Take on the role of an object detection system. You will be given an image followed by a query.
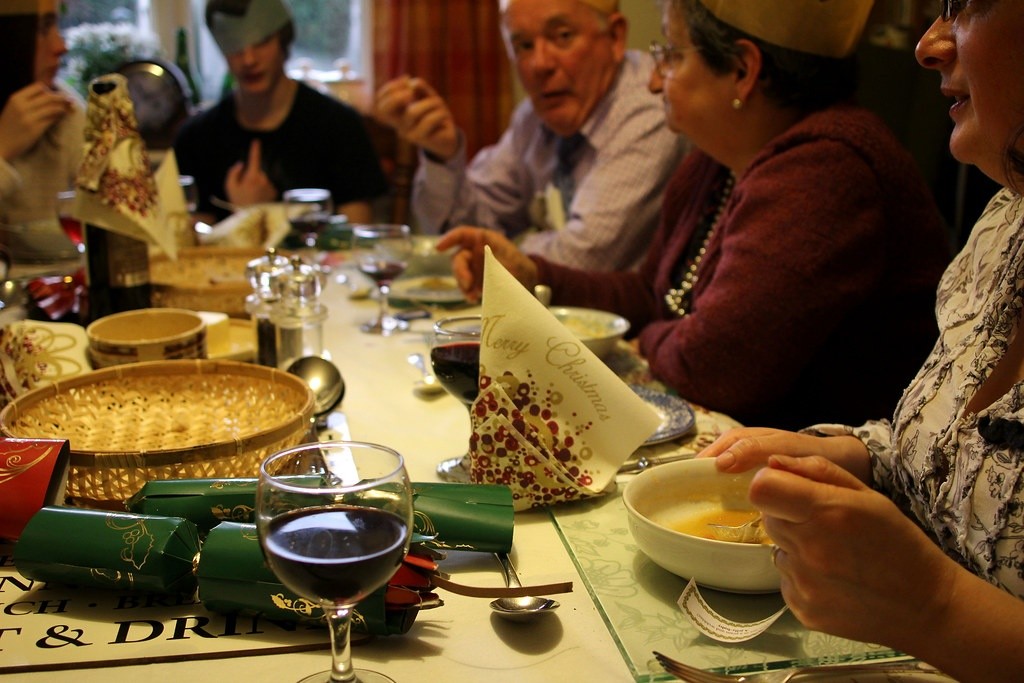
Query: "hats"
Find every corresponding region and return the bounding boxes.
[702,1,877,59]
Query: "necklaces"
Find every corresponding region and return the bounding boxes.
[664,174,740,319]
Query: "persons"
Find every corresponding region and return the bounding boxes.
[377,0,700,274]
[0,0,89,264]
[435,0,954,434]
[693,0,1024,683]
[171,0,388,232]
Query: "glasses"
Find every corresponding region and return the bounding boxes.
[941,0,968,22]
[649,39,742,78]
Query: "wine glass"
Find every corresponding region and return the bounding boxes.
[431,316,482,482]
[351,224,411,333]
[284,190,330,271]
[255,442,414,683]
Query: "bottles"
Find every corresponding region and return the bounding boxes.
[85,79,154,318]
[244,250,328,430]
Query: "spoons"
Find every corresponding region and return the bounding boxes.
[707,517,769,544]
[286,356,345,414]
[490,552,560,623]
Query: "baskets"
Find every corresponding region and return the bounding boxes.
[148,252,304,316]
[1,358,316,512]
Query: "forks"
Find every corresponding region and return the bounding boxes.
[651,650,940,683]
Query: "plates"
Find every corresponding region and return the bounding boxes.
[630,386,694,447]
[371,275,466,305]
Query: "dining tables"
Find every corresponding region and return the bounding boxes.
[0,236,956,683]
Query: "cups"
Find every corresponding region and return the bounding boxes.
[180,174,198,215]
[54,192,84,251]
[86,307,207,370]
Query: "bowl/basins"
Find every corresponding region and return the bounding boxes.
[545,306,631,361]
[622,459,783,595]
[377,236,459,274]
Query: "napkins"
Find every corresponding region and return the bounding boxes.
[472,244,664,513]
[0,321,100,405]
[74,75,175,260]
